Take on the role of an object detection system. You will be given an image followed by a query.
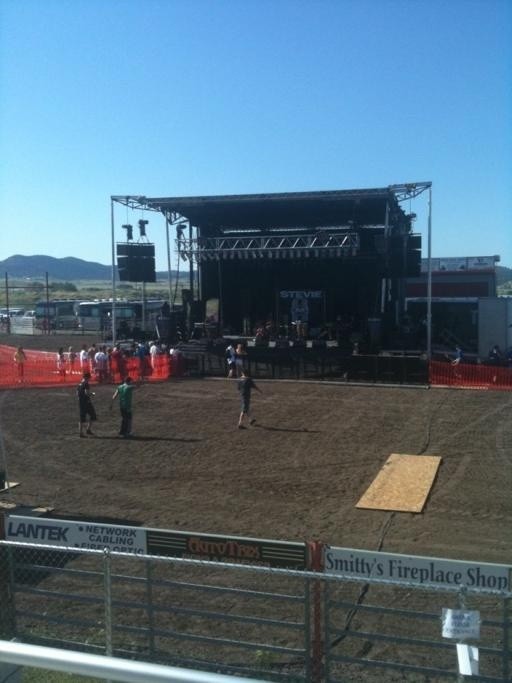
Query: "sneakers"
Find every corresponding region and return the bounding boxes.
[239,425,247,429]
[250,419,256,425]
[80,432,87,438]
[86,430,94,434]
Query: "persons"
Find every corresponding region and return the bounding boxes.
[110,375,141,439]
[237,369,263,429]
[76,372,96,438]
[224,319,276,380]
[13,337,185,384]
[453,345,508,366]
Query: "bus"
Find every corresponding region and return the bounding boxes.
[35,298,171,332]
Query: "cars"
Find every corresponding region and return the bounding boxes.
[0,307,36,324]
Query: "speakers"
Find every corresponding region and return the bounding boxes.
[117,244,156,283]
[375,236,422,279]
